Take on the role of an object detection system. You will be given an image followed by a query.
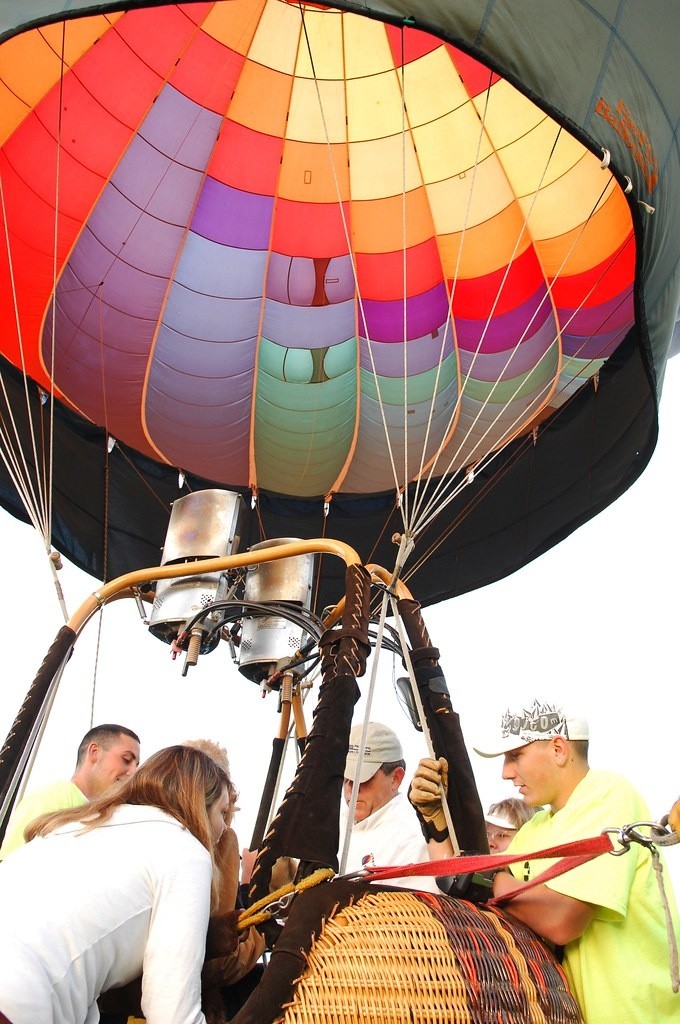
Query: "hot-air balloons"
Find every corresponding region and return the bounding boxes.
[4,0,680,1024]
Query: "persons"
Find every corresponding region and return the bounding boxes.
[0,745,235,1024]
[0,696,680,1024]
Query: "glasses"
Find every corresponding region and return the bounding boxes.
[486,830,511,838]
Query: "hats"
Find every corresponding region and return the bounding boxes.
[484,806,518,829]
[344,722,402,783]
[474,673,590,758]
